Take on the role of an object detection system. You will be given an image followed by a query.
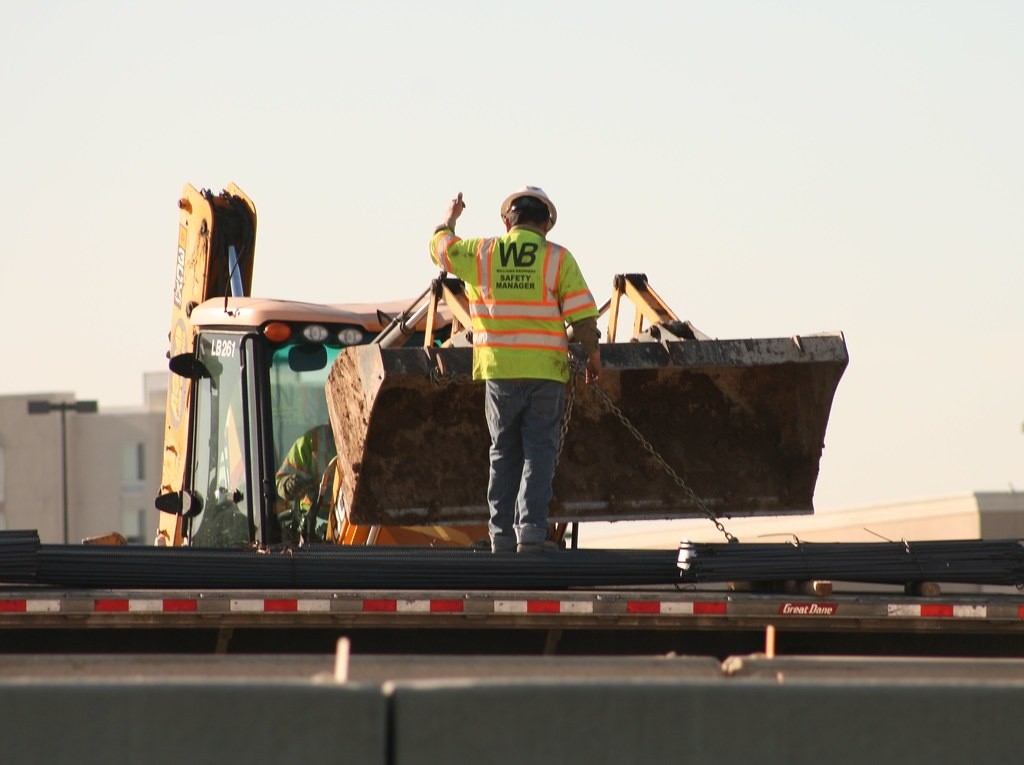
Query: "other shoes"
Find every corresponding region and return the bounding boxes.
[496,538,559,555]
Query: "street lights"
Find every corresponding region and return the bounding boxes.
[27,398,98,543]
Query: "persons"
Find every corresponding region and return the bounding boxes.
[427,183,605,556]
[275,417,337,541]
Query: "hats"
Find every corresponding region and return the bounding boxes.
[501,186,557,233]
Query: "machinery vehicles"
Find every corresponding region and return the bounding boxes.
[153,183,850,553]
